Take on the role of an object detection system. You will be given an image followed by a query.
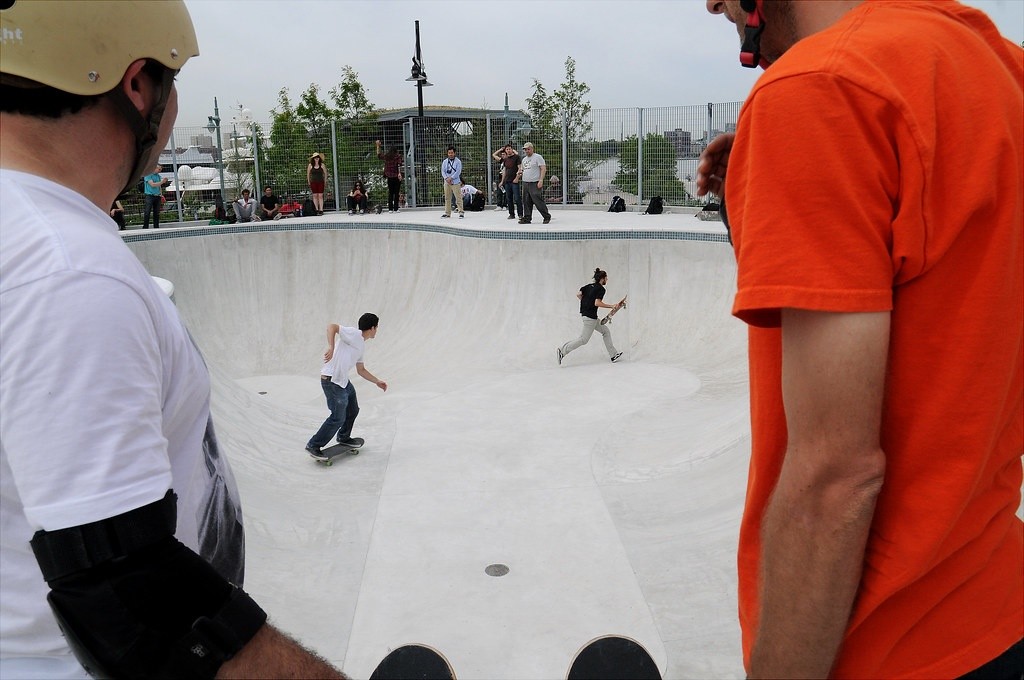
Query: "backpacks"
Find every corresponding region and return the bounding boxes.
[302,198,317,217]
[645,196,663,215]
[607,195,626,212]
[471,192,485,211]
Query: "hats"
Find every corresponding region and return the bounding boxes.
[309,152,325,163]
[522,142,533,150]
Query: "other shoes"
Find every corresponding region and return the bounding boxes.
[494,205,502,210]
[507,215,515,219]
[441,214,450,218]
[518,218,532,224]
[459,215,464,218]
[395,209,400,213]
[519,216,524,220]
[543,216,551,224]
[235,210,392,224]
[502,207,506,210]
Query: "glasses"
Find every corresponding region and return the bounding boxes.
[355,185,361,187]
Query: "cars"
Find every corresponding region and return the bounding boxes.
[194,205,216,220]
[161,203,191,219]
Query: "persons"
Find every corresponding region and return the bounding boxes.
[305,312,388,460]
[109,164,172,231]
[347,182,369,215]
[545,174,564,204]
[555,268,627,366]
[492,142,552,225]
[440,148,482,219]
[231,185,301,223]
[307,152,328,213]
[1,0,353,679]
[375,139,403,214]
[698,1,1024,680]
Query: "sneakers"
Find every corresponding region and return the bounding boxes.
[610,352,623,362]
[304,445,329,460]
[336,437,361,447]
[557,348,563,364]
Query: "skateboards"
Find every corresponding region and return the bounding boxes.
[369,641,458,680]
[309,437,367,466]
[565,633,663,680]
[282,214,294,219]
[215,193,225,220]
[600,294,628,325]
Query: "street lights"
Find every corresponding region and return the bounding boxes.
[405,20,433,205]
[504,92,532,146]
[203,97,228,217]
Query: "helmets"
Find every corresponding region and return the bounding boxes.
[0,0,200,135]
